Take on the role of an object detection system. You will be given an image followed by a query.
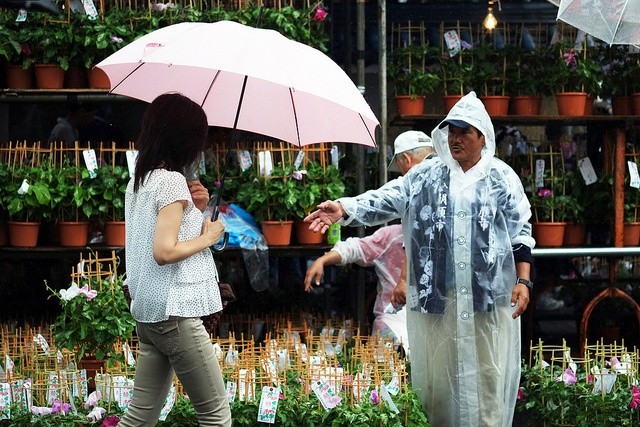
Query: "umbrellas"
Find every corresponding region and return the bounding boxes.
[92,20,381,254]
[555,0,640,48]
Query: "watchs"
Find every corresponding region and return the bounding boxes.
[514,277,535,289]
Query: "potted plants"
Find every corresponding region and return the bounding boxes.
[387,34,428,115]
[628,57,640,115]
[600,51,632,116]
[0,168,9,248]
[29,14,64,89]
[477,55,510,115]
[253,169,294,245]
[605,168,640,246]
[58,14,88,87]
[1,11,34,89]
[561,165,587,247]
[435,42,472,110]
[85,18,114,89]
[507,48,543,116]
[92,166,126,246]
[55,166,89,246]
[9,169,51,247]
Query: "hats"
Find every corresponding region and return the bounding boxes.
[438,120,471,131]
[388,130,433,173]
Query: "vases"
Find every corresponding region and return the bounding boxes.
[296,219,326,244]
[537,222,563,246]
[558,90,590,116]
[78,356,115,391]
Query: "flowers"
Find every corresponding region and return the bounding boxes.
[528,175,559,220]
[552,48,590,90]
[49,277,136,359]
[296,164,336,213]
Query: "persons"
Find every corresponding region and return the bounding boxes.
[116,92,234,427]
[302,90,536,427]
[302,224,409,366]
[387,129,438,310]
